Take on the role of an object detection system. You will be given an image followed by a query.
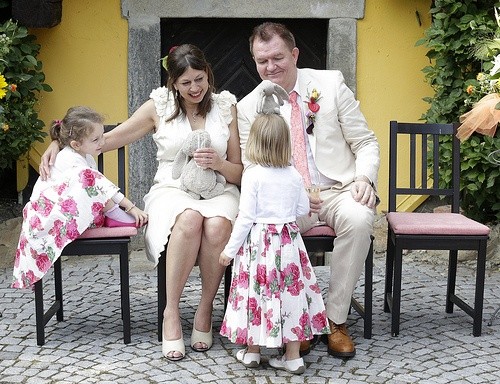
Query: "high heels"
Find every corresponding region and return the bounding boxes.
[190,311,212,352]
[161,318,185,360]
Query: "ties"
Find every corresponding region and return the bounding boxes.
[285,92,311,188]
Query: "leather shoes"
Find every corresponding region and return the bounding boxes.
[300,333,319,355]
[321,319,356,356]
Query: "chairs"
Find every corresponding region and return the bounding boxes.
[34,122,136,345]
[384,121,490,336]
[302,226,375,339]
[158,238,232,341]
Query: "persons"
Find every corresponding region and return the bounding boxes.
[218,113,331,374]
[237,22,380,360]
[39,43,244,360]
[11,105,149,292]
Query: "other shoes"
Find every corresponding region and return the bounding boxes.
[236,347,261,367]
[269,354,305,374]
[103,216,149,228]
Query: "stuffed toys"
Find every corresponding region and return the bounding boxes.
[171,129,226,200]
[255,80,290,115]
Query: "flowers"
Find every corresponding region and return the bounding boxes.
[304,90,323,133]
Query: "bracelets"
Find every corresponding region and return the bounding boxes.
[125,201,136,213]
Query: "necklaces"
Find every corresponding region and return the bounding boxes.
[188,109,198,121]
